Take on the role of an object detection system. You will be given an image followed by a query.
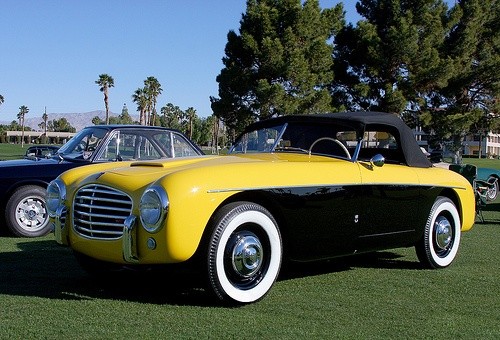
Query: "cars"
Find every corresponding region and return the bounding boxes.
[43,111,476,310]
[23,145,61,159]
[0,125,207,238]
[435,162,500,202]
